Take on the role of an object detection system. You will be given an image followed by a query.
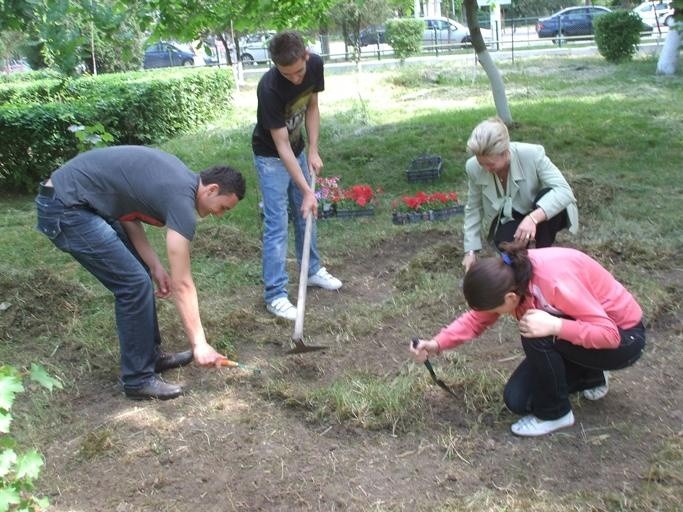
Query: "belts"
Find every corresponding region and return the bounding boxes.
[39,182,54,197]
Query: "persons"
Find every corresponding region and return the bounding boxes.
[459,115,579,276]
[407,239,646,438]
[249,30,342,322]
[37,144,247,401]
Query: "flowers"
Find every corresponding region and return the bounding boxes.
[391,191,461,211]
[333,185,374,209]
[314,174,341,210]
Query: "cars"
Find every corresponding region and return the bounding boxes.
[240,31,319,65]
[415,18,491,50]
[348,26,391,47]
[626,0,675,26]
[535,5,653,45]
[143,43,194,68]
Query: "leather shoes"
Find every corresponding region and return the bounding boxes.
[155,351,193,373]
[511,410,574,436]
[584,370,609,399]
[124,378,181,399]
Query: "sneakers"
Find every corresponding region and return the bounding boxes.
[307,267,342,290]
[266,297,297,320]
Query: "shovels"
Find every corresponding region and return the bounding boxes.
[412,337,460,400]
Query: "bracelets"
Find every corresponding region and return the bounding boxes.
[465,249,476,256]
[528,213,539,226]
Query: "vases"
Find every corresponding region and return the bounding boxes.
[337,208,375,217]
[318,209,334,218]
[392,206,464,224]
